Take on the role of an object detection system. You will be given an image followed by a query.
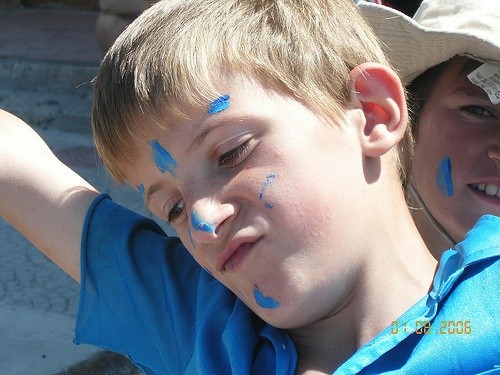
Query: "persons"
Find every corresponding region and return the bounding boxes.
[398,0,500,259]
[0,0,499,375]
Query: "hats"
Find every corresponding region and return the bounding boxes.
[355,0,500,89]
